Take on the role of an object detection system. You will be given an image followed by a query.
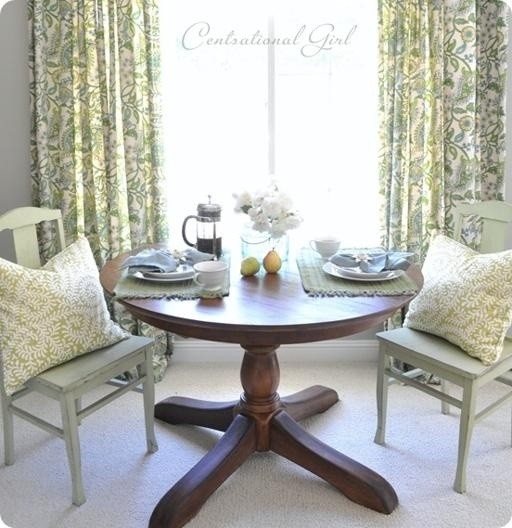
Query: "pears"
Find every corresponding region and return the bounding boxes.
[240,256,260,277]
[263,247,282,274]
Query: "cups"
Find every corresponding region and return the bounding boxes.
[192,260,229,292]
[308,236,341,260]
[181,194,224,258]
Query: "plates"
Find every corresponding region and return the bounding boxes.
[321,258,403,282]
[129,264,194,282]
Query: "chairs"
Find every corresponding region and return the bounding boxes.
[373,200,511,495]
[0,206,160,510]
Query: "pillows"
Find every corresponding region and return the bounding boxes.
[402,228,511,366]
[0,234,131,398]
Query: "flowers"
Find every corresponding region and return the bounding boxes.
[231,186,304,239]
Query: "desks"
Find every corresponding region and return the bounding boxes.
[98,241,425,527]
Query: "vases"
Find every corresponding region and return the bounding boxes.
[241,232,289,270]
